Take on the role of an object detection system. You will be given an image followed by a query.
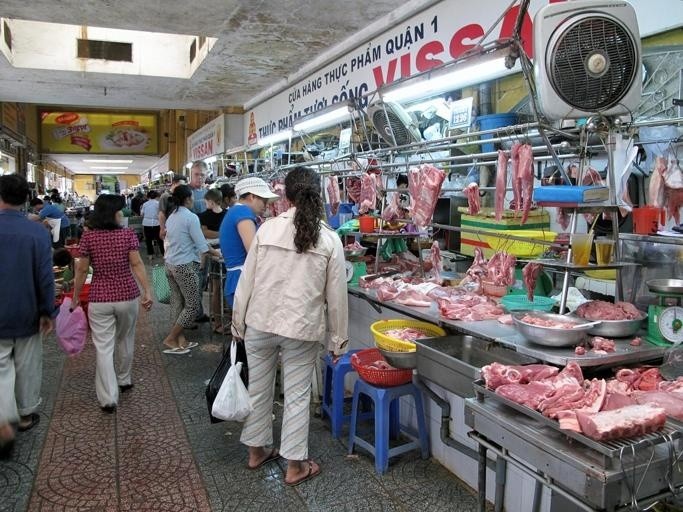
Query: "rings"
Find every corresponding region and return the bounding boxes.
[148,306,150,309]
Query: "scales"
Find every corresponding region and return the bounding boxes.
[643,278,683,347]
[507,256,553,296]
[344,246,367,288]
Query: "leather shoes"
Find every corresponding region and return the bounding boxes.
[119,384,133,392]
[102,405,114,414]
[194,314,232,335]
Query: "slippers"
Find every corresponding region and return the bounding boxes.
[245,447,281,469]
[163,341,199,355]
[18,412,40,430]
[284,461,321,487]
[0,439,14,459]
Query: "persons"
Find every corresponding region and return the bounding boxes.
[189,162,234,333]
[71,194,154,415]
[162,185,209,354]
[1,175,56,460]
[127,176,186,260]
[32,189,70,248]
[219,176,276,422]
[230,166,350,487]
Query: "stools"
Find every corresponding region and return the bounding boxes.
[319,349,374,439]
[347,378,430,475]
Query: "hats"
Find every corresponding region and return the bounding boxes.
[234,177,282,205]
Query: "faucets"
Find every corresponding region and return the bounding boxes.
[485,339,500,352]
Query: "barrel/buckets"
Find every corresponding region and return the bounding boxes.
[61,284,91,329]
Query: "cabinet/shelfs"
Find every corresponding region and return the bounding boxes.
[208,248,232,358]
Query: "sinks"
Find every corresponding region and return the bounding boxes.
[411,334,542,399]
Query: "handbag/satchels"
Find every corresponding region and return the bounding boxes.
[152,265,172,304]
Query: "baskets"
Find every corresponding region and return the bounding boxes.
[370,320,447,353]
[350,346,412,387]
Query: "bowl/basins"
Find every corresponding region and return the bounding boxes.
[373,345,417,370]
[512,311,594,347]
[572,311,648,339]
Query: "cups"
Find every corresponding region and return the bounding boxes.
[359,216,378,233]
[594,239,615,266]
[572,233,595,267]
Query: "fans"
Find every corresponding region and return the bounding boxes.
[532,0,641,123]
[366,98,421,147]
